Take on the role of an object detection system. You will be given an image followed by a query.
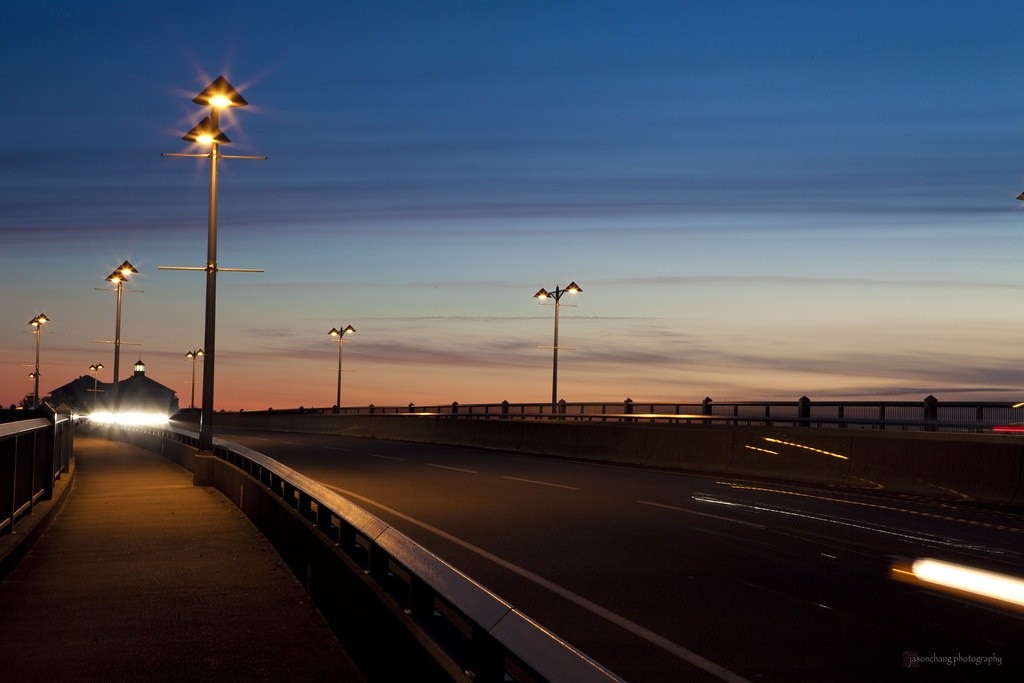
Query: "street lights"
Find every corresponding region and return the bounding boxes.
[186,73,249,450]
[533,281,584,414]
[328,325,357,413]
[28,313,50,409]
[89,362,105,413]
[186,347,204,410]
[105,259,140,414]
